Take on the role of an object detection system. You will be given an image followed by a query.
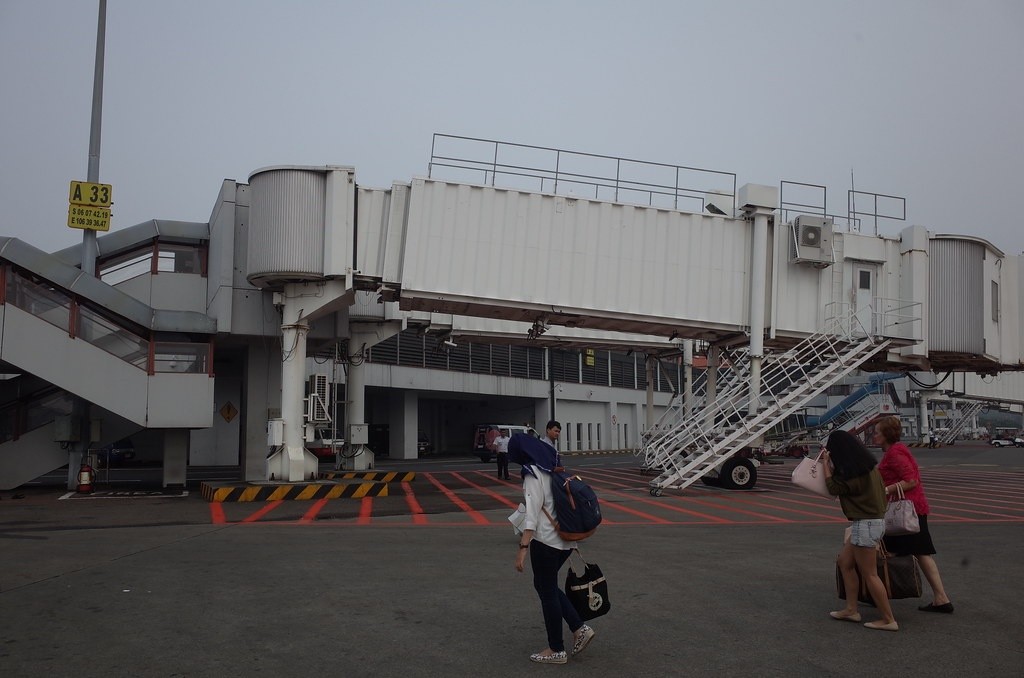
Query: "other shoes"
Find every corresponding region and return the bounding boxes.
[529,650,567,664]
[918,602,954,614]
[505,477,512,480]
[864,622,899,631]
[571,625,594,656]
[497,476,504,479]
[830,611,861,622]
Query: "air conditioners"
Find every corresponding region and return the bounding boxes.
[792,215,833,268]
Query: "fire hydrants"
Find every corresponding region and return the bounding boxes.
[77,463,97,495]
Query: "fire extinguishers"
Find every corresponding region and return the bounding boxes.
[77,461,96,493]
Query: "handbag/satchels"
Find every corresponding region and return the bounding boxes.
[877,538,922,600]
[885,482,920,535]
[791,450,838,500]
[835,554,877,607]
[565,548,611,622]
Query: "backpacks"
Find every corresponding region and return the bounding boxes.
[542,465,602,541]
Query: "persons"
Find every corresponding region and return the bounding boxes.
[826,429,900,632]
[541,420,562,448]
[492,429,511,480]
[873,417,953,614]
[507,433,596,664]
[929,427,935,450]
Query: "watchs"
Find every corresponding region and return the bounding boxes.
[519,542,528,551]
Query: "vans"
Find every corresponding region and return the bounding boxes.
[370,423,432,457]
[304,428,344,461]
[935,427,951,440]
[473,425,543,463]
